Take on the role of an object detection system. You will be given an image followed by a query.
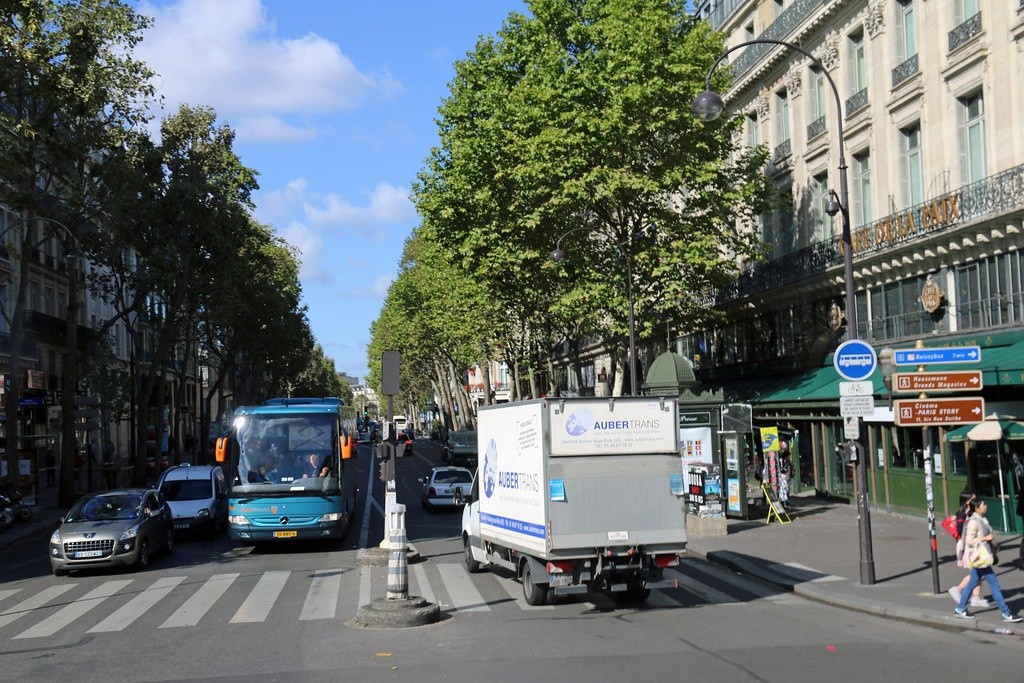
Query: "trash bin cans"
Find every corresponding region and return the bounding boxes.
[57,468,80,505]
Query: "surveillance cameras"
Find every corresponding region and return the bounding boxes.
[825,201,840,216]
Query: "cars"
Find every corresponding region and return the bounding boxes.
[401,429,415,441]
[441,430,477,468]
[397,433,414,457]
[417,466,473,516]
[48,488,174,577]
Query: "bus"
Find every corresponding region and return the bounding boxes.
[392,415,407,432]
[215,396,359,549]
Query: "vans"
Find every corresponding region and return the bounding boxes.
[150,463,226,538]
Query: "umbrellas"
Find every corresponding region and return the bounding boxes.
[943,412,1024,534]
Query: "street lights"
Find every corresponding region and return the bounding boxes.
[549,226,638,394]
[129,300,189,467]
[691,38,876,586]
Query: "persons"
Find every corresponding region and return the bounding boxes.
[257,465,274,482]
[948,490,989,608]
[184,435,194,453]
[74,450,83,480]
[781,451,796,508]
[302,453,329,478]
[954,498,1024,624]
[168,435,174,456]
[91,439,96,463]
[107,440,112,461]
[44,450,57,488]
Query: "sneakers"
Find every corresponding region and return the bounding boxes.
[1001,612,1024,623]
[953,607,975,620]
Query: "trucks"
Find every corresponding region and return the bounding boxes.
[452,394,688,606]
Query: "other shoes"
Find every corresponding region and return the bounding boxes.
[948,585,963,604]
[969,597,991,607]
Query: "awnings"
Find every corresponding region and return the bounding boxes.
[746,332,1024,420]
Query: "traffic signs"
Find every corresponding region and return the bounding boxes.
[894,395,985,427]
[892,345,983,365]
[891,369,984,395]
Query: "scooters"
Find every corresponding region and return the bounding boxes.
[0,483,32,529]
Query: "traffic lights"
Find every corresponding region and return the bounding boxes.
[364,405,367,415]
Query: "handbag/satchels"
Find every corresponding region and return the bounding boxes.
[956,540,1002,571]
[939,513,967,540]
